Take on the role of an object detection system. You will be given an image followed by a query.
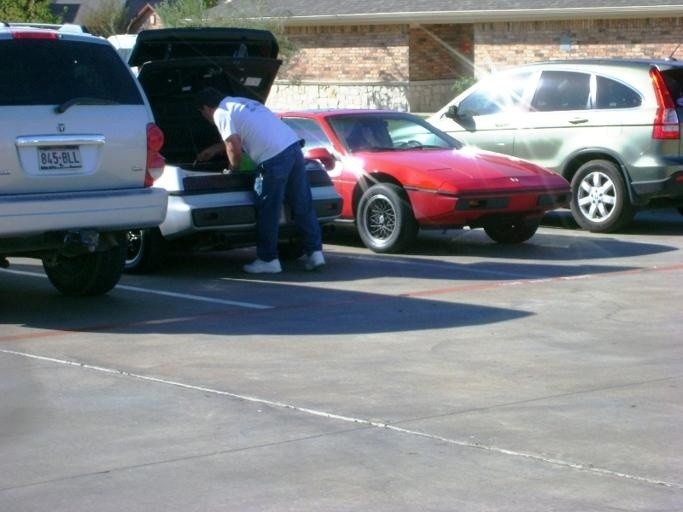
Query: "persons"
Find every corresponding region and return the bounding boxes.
[194,87,325,274]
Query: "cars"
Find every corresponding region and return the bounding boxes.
[276,108,573,254]
[389,58,683,234]
[107,27,344,274]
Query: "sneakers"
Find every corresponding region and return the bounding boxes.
[242,258,281,273]
[304,251,326,271]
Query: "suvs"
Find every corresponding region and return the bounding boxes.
[0,20,169,296]
